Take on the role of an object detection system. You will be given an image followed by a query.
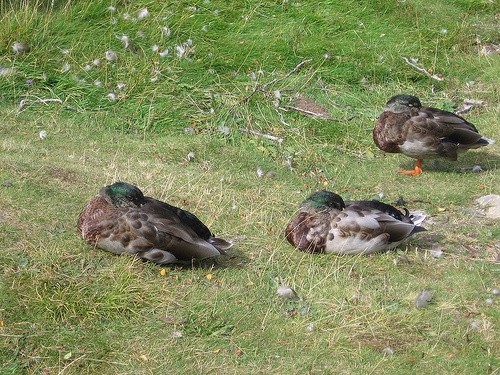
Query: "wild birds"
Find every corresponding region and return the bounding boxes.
[373,94,490,175]
[75,181,234,265]
[282,189,426,255]
